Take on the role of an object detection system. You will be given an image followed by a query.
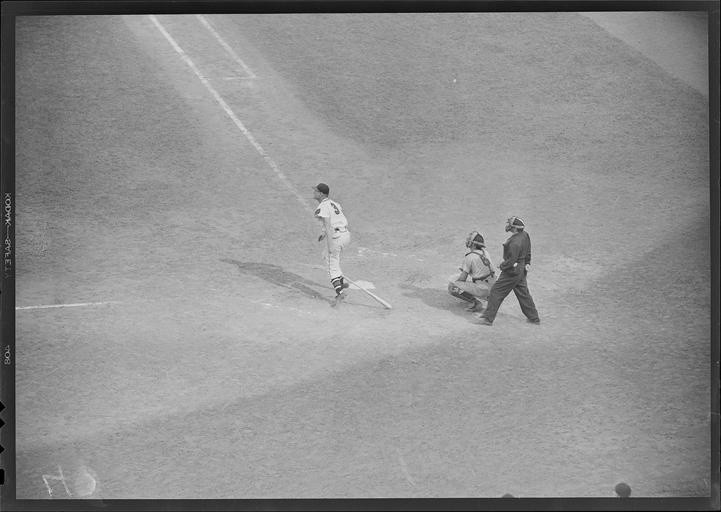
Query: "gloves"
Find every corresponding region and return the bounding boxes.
[318,233,325,241]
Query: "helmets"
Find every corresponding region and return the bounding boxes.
[505,216,525,232]
[466,231,485,247]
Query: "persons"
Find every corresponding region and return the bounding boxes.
[613,479,633,498]
[446,215,542,326]
[310,182,352,299]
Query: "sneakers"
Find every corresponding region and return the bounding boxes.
[465,300,493,327]
[335,283,350,298]
[526,318,540,325]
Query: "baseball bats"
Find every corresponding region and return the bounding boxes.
[343,276,392,309]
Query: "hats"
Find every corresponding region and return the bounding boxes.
[311,183,329,194]
